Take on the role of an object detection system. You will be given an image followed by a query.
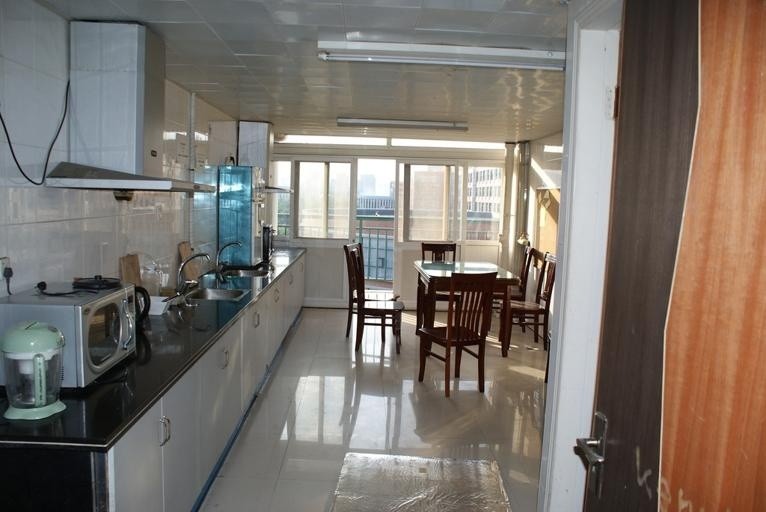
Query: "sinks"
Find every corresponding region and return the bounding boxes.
[185,287,252,301]
[224,269,270,279]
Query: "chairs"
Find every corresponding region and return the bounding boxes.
[343,242,400,343]
[499,252,556,351]
[418,272,498,397]
[350,247,404,354]
[422,243,461,328]
[490,245,535,333]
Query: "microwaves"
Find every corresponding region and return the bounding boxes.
[0,279,139,390]
[264,224,275,258]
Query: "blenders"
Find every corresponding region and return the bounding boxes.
[0,320,70,423]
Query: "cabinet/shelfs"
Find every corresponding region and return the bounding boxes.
[240,292,268,410]
[263,275,285,367]
[200,319,243,490]
[283,251,305,337]
[105,360,200,512]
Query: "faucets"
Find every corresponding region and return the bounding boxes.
[215,241,242,270]
[177,253,211,288]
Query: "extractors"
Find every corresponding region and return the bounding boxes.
[42,19,216,194]
[237,119,294,195]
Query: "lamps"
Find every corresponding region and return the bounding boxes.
[317,40,566,71]
[336,116,468,131]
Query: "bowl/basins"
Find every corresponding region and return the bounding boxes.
[147,314,174,334]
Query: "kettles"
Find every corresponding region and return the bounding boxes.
[133,332,151,367]
[134,284,151,331]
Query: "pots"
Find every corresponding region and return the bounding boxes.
[141,296,175,316]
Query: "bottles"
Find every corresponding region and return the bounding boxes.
[141,263,163,297]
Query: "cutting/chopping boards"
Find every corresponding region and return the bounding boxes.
[177,240,200,281]
[119,254,143,290]
